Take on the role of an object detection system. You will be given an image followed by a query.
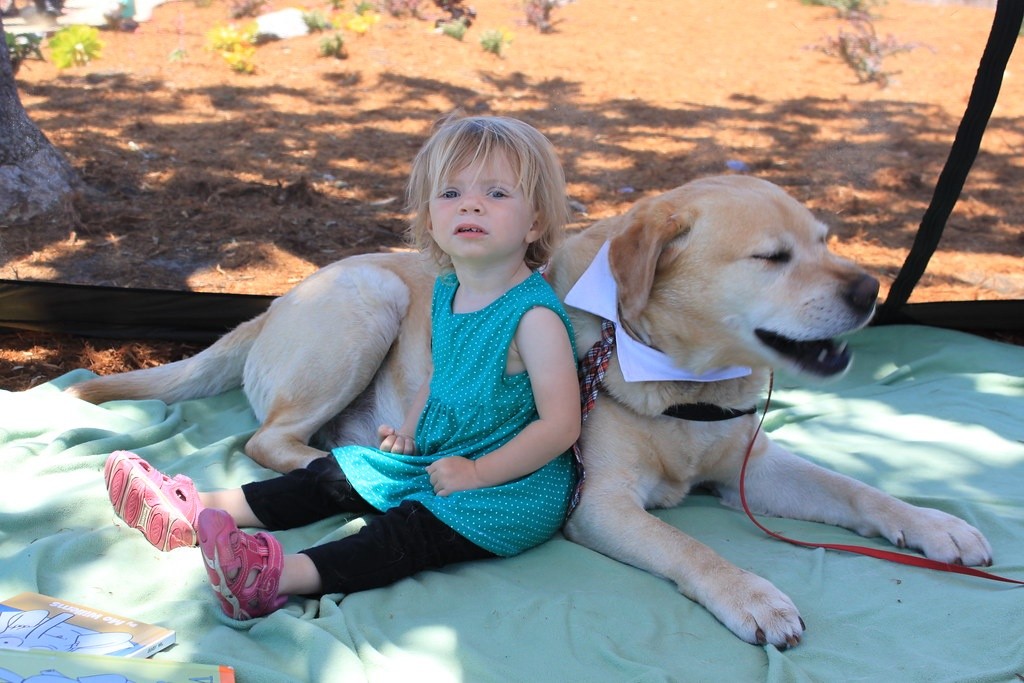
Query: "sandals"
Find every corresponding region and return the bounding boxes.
[104,450,204,552]
[198,508,288,621]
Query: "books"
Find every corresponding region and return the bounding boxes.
[0,590,235,683]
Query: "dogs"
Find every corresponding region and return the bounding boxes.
[66,174,996,652]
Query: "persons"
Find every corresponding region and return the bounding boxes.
[103,104,582,621]
